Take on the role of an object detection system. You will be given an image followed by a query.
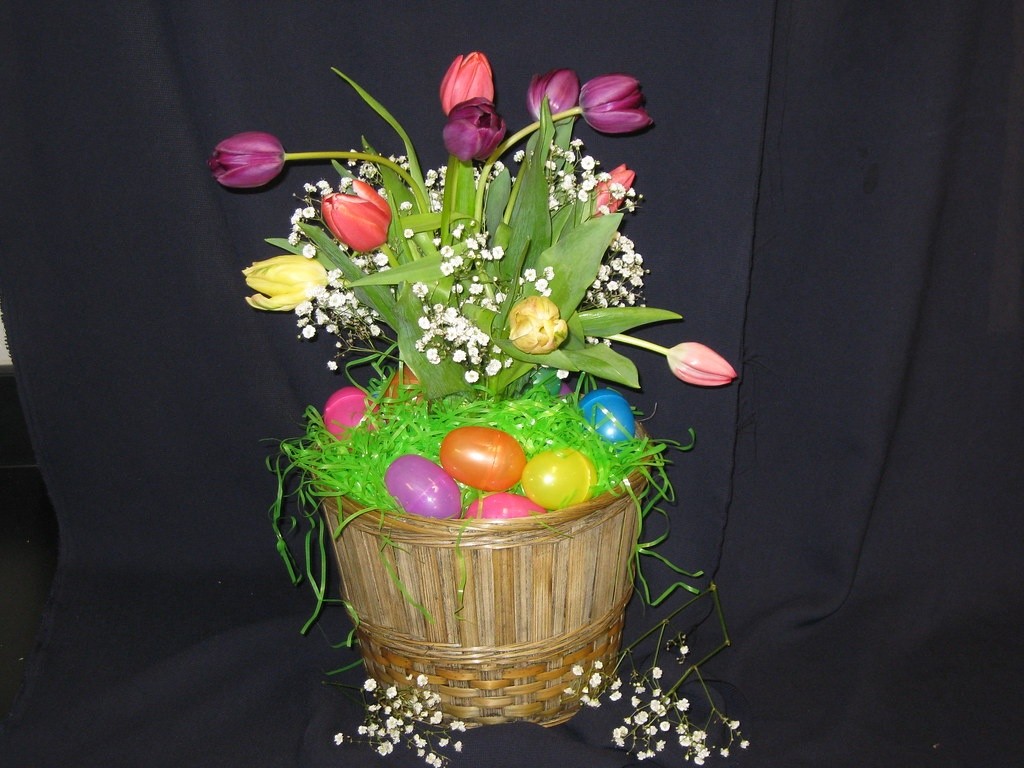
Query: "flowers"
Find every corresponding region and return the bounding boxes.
[558,579,753,764]
[201,48,742,406]
[306,665,473,767]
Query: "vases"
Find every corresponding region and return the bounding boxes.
[296,418,656,724]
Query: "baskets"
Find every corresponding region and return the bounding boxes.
[310,419,655,727]
